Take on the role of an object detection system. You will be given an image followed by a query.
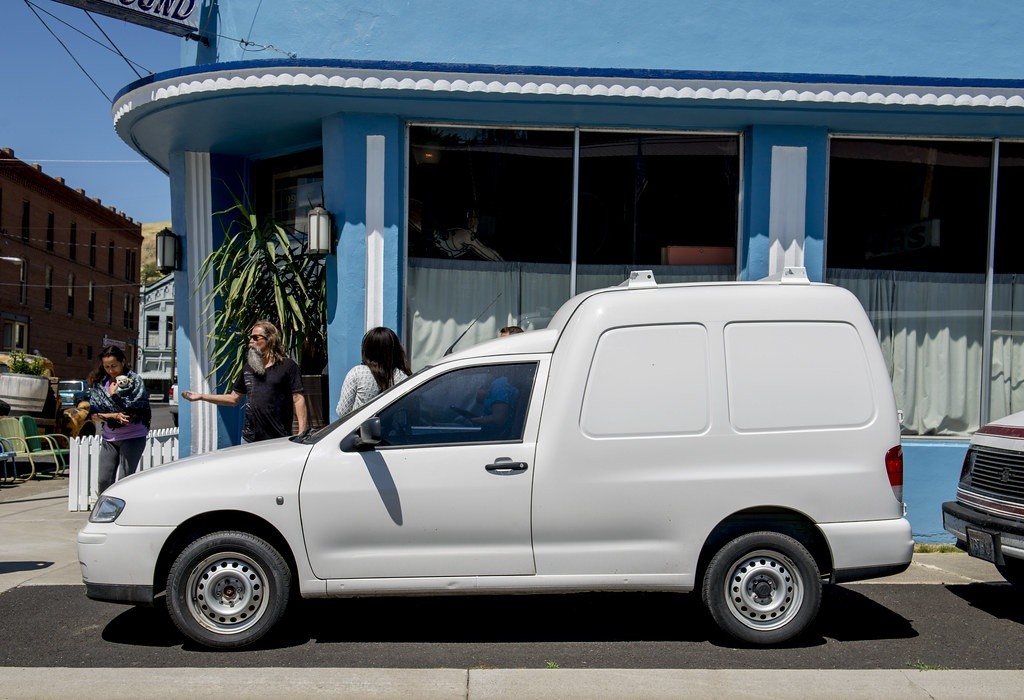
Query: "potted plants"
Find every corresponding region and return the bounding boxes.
[190,196,329,436]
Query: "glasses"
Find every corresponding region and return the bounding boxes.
[247,335,268,341]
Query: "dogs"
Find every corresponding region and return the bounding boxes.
[114,375,134,393]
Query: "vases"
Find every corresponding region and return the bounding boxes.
[0,373,48,411]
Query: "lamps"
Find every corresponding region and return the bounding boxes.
[307,206,335,257]
[155,227,183,274]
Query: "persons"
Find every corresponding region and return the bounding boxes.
[182,321,307,446]
[87,345,151,506]
[471,361,519,442]
[500,326,524,339]
[336,326,408,419]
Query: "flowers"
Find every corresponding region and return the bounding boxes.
[9,350,54,376]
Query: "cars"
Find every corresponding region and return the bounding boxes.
[77,269,918,650]
[57,379,93,407]
[169,383,178,427]
[942,410,1024,593]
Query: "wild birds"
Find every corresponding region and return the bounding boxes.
[56,401,91,440]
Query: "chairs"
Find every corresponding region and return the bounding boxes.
[505,372,535,440]
